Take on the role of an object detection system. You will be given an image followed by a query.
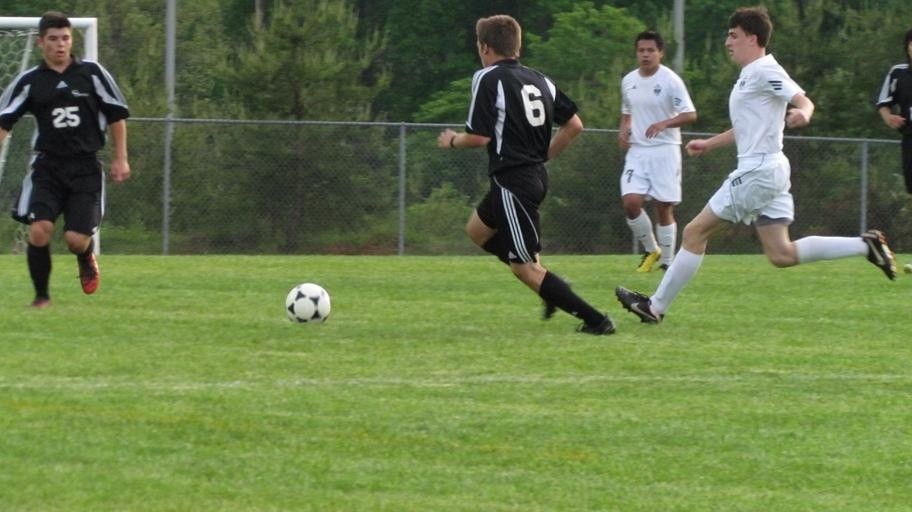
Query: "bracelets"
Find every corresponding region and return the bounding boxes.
[448,130,459,148]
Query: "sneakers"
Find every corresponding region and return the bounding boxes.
[31,299,50,307]
[636,247,661,273]
[77,252,100,294]
[615,286,664,323]
[577,316,617,335]
[860,229,897,280]
[542,300,556,320]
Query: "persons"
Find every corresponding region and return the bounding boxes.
[614,5,899,328]
[436,13,614,337]
[877,26,910,191]
[614,28,701,279]
[2,9,132,310]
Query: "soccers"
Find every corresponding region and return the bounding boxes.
[285,282,332,325]
